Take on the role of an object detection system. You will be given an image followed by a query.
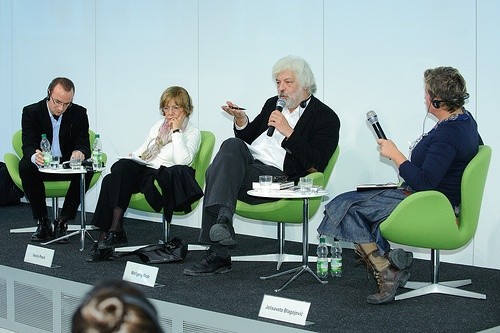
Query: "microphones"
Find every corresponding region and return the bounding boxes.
[366,111,388,140]
[267,99,286,137]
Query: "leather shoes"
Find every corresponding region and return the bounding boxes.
[53,219,71,244]
[30,219,53,242]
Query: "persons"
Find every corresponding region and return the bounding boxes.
[17,78,94,243]
[183,56,340,276]
[71,280,163,333]
[316,66,483,305]
[90,87,201,256]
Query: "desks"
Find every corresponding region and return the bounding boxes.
[247,189,330,292]
[39,164,99,252]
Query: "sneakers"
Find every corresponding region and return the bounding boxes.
[183,249,233,276]
[209,215,237,246]
[98,230,128,249]
[86,243,113,262]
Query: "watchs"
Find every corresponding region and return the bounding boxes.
[172,129,182,133]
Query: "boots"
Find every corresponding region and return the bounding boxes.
[354,242,411,304]
[384,248,413,270]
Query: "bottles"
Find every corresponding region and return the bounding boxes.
[40,134,52,169]
[317,238,329,279]
[330,238,343,278]
[92,133,103,171]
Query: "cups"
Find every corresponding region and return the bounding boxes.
[259,176,272,191]
[49,154,59,170]
[71,155,81,169]
[299,177,313,195]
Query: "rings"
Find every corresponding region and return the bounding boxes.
[173,118,175,120]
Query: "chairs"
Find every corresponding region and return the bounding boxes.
[380,146,491,300]
[4,130,106,233]
[114,130,214,252]
[230,142,341,262]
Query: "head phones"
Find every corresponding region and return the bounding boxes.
[299,95,313,109]
[432,93,470,109]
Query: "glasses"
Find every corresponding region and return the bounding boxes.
[162,106,182,112]
[50,95,72,107]
